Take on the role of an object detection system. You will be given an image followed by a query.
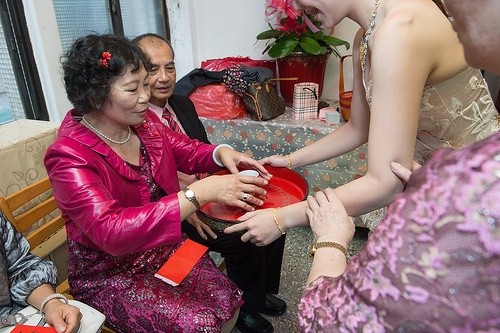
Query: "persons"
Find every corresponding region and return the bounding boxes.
[0,208,83,333]
[44,29,273,333]
[297,0,500,333]
[224,0,500,246]
[132,33,287,333]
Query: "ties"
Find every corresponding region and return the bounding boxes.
[162,110,185,137]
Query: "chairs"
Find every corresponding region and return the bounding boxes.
[0,178,116,333]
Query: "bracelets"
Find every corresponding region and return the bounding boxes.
[212,144,234,167]
[273,206,285,234]
[40,293,68,317]
[286,154,292,170]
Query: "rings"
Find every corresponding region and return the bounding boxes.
[243,193,249,201]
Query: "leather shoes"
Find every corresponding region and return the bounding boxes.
[254,292,288,317]
[236,306,274,333]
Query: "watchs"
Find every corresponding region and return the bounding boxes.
[309,242,349,264]
[183,186,200,209]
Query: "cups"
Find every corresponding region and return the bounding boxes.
[237,170,259,196]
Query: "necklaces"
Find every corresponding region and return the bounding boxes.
[360,0,381,72]
[82,114,131,144]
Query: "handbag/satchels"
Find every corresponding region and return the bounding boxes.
[292,82,319,120]
[242,76,299,121]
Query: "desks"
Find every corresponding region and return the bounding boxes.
[0,119,67,305]
[196,98,434,228]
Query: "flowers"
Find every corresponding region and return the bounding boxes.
[254,0,350,59]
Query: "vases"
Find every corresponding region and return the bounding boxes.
[277,55,327,108]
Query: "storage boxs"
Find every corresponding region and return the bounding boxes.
[326,111,341,124]
